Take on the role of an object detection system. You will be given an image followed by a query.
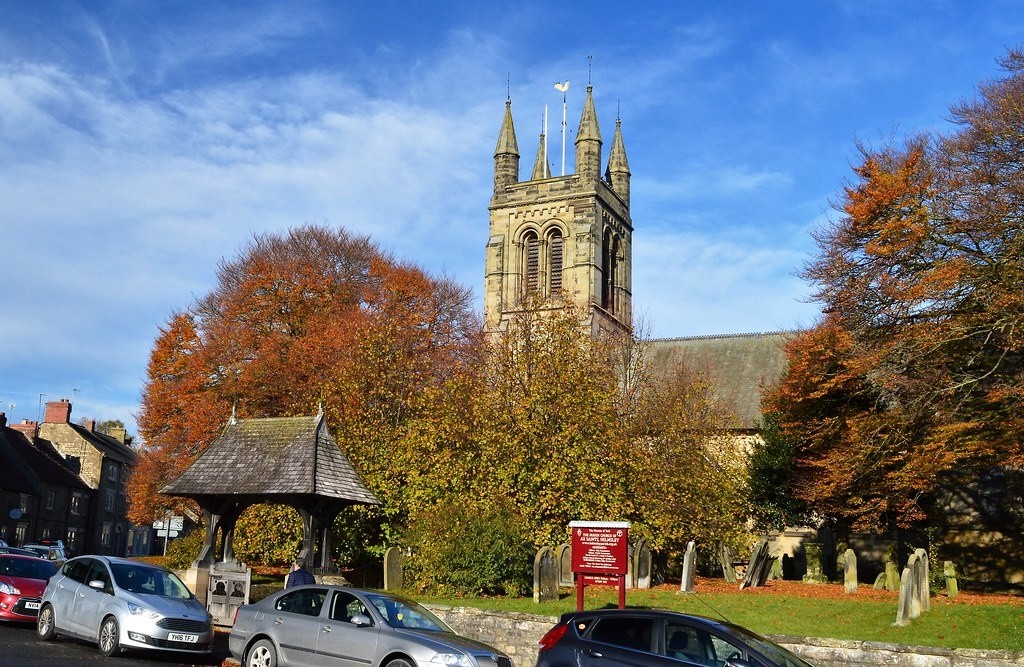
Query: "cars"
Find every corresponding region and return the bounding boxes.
[0,554,58,621]
[0,546,37,556]
[536,608,819,667]
[20,543,66,560]
[36,537,65,552]
[0,540,8,547]
[228,584,516,666]
[37,554,214,656]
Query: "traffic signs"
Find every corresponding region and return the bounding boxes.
[154,509,184,538]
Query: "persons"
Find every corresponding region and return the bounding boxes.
[286,557,321,610]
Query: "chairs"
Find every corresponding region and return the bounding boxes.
[134,573,153,593]
[364,606,379,624]
[668,630,693,661]
[334,601,351,623]
